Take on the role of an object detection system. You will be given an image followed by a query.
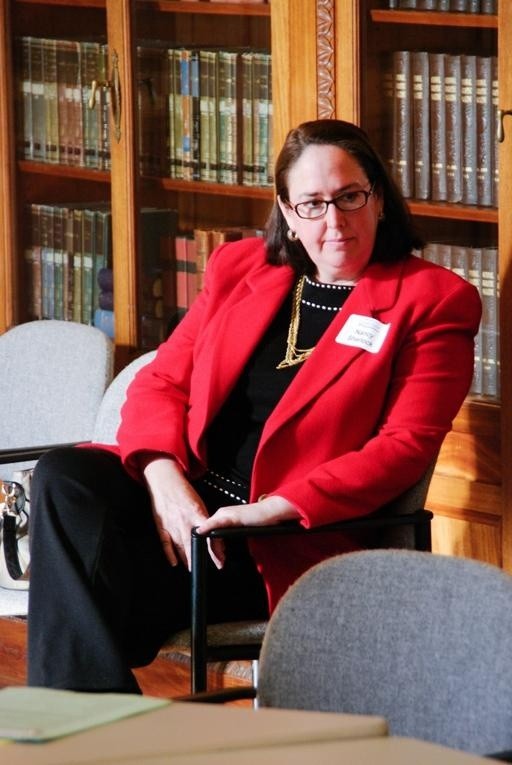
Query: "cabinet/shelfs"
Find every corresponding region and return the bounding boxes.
[0,0,311,381]
[311,2,511,573]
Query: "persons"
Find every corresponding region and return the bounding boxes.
[27,120,481,693]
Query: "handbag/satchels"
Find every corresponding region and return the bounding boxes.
[0,464,40,593]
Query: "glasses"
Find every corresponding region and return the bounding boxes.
[281,184,379,220]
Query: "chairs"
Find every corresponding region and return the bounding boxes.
[1,319,167,575]
[154,420,448,695]
[173,548,511,763]
